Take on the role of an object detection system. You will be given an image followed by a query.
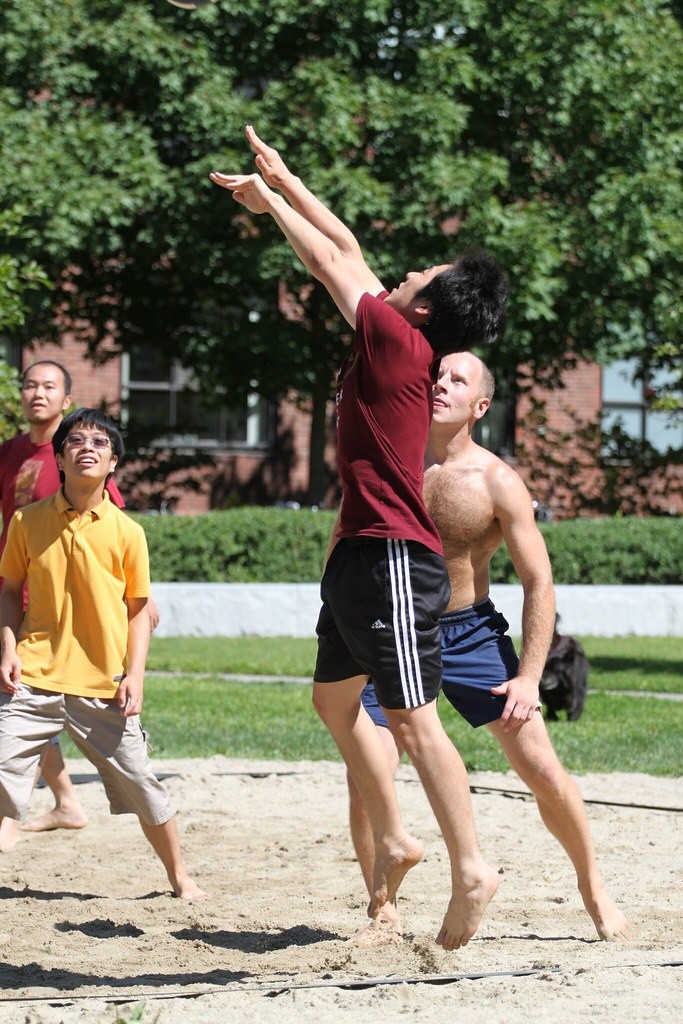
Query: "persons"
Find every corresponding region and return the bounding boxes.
[1,407,194,903]
[0,361,160,832]
[210,124,505,951]
[348,351,628,940]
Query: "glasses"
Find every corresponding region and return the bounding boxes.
[61,435,116,452]
